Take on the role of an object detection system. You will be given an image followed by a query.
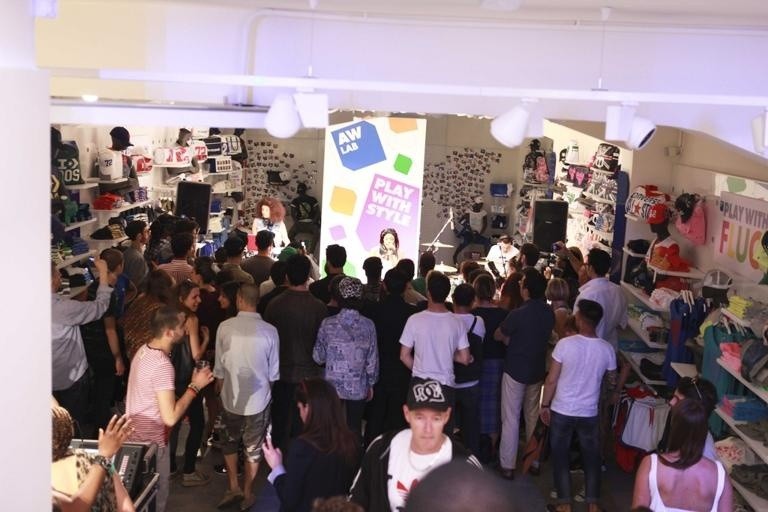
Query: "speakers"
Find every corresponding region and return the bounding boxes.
[174,180,212,234]
[532,198,569,252]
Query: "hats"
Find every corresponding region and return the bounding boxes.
[474,197,484,204]
[131,145,153,160]
[559,164,588,189]
[383,263,410,289]
[180,128,191,134]
[693,270,733,291]
[675,194,697,223]
[109,126,135,146]
[209,128,221,135]
[645,203,669,226]
[51,128,67,151]
[407,378,448,412]
[339,277,363,299]
[528,140,541,146]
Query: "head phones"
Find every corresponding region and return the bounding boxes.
[263,217,273,227]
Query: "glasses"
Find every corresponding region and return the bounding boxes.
[300,376,309,397]
[692,375,702,399]
[518,280,523,287]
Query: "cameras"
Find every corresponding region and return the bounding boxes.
[197,360,210,372]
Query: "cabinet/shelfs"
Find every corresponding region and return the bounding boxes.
[50,151,242,301]
[514,167,768,512]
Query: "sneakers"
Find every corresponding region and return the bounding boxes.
[546,458,586,511]
[217,488,244,510]
[206,433,221,449]
[182,471,215,486]
[167,463,184,483]
[214,464,245,480]
[240,493,256,511]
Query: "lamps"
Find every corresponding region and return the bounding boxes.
[608,102,656,151]
[486,105,535,150]
[264,90,303,141]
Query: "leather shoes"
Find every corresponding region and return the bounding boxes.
[518,458,540,476]
[489,461,514,481]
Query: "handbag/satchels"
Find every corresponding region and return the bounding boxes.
[593,144,620,172]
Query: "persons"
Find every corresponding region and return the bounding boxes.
[230,128,248,163]
[206,128,222,173]
[454,196,491,264]
[167,126,199,176]
[632,204,690,294]
[99,126,140,196]
[51,125,69,213]
[522,139,543,172]
[251,197,290,259]
[287,182,320,263]
[51,219,768,511]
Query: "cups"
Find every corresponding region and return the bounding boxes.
[196,359,210,373]
[154,148,166,164]
